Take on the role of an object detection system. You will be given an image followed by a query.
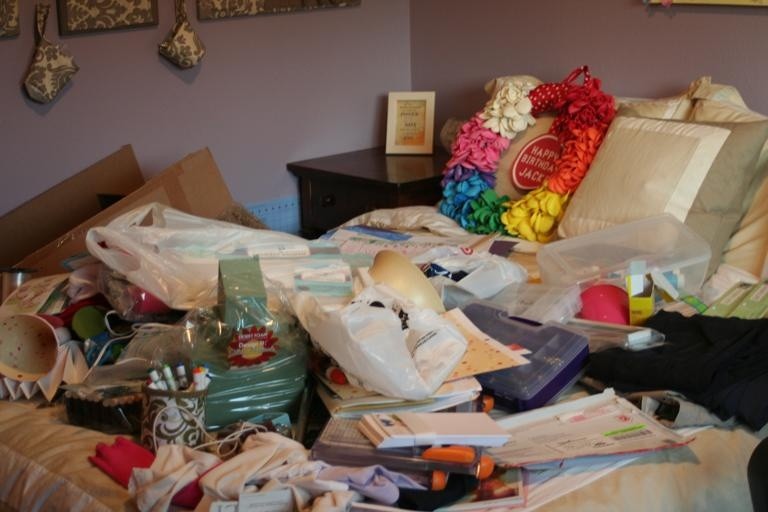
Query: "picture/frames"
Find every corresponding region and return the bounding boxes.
[384,90,436,157]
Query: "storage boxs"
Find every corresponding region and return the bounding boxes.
[2,145,268,278]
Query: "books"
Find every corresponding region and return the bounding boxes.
[311,372,511,475]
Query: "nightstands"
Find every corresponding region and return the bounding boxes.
[286,147,453,241]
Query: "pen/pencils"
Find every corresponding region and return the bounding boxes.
[146,361,212,392]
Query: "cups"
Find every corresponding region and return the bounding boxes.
[138,382,210,457]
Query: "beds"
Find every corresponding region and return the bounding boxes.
[2,98,768,512]
